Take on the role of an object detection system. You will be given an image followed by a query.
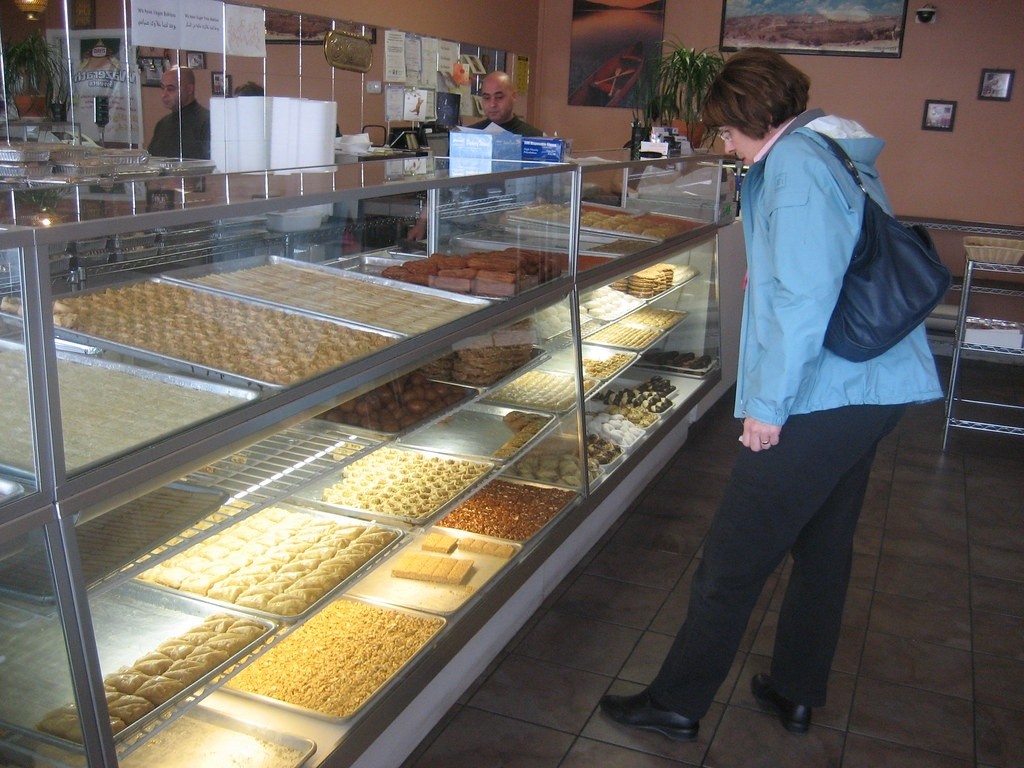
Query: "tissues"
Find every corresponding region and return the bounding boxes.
[449,121,522,162]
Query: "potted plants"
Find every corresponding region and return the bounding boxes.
[637,29,726,148]
[2,28,69,117]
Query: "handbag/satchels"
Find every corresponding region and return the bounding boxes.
[824,194,954,363]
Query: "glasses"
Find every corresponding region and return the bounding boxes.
[717,125,728,141]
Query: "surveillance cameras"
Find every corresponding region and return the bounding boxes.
[916,8,936,23]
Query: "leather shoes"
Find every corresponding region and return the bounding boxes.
[751,671,812,735]
[600,689,700,743]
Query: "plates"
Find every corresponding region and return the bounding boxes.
[500,377,677,489]
[630,363,718,378]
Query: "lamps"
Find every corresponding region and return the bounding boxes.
[14,0,49,20]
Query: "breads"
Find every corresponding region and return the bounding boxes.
[0,203,718,751]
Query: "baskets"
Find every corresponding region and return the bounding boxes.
[962,236,1024,264]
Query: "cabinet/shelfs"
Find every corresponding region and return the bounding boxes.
[943,260,1024,450]
[896,215,1024,366]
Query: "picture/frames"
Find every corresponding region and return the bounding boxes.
[922,99,957,131]
[978,69,1014,101]
[211,72,232,98]
[719,0,908,58]
[71,0,96,30]
[185,51,207,69]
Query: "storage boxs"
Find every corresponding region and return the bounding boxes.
[650,125,691,154]
[450,127,566,164]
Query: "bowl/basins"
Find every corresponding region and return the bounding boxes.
[338,142,373,152]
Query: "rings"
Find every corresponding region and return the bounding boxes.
[760,439,770,444]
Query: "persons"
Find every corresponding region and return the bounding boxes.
[144,65,264,190]
[408,71,549,242]
[601,50,945,742]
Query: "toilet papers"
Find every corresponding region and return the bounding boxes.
[207,95,273,175]
[269,92,337,175]
